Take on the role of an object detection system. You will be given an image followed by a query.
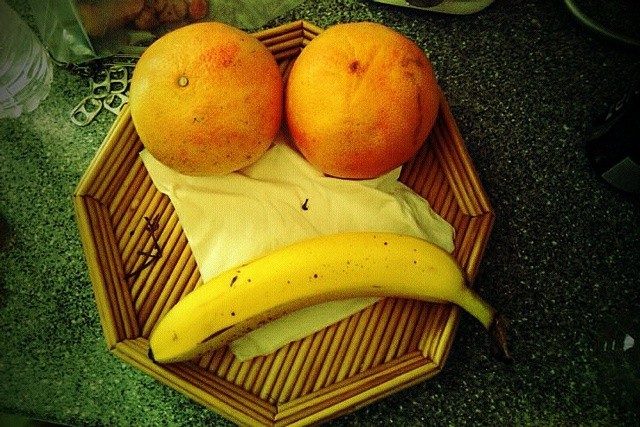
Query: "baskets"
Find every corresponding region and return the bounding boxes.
[74,19,496,427]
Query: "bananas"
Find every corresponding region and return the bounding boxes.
[147,232,507,365]
